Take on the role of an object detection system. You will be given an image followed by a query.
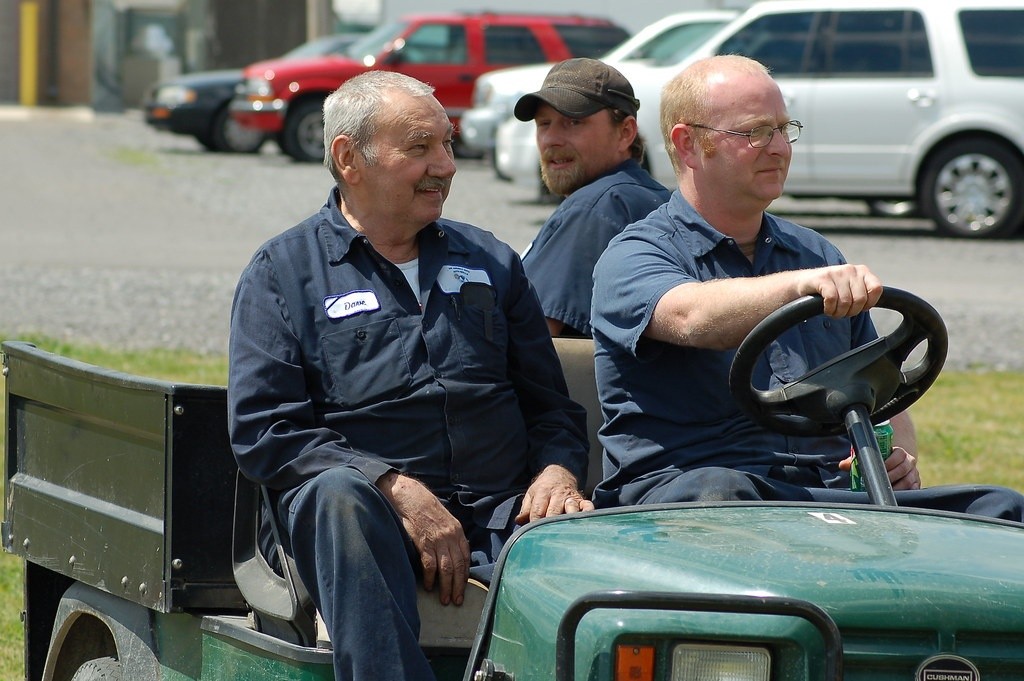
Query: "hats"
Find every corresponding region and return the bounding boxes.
[514,58,637,122]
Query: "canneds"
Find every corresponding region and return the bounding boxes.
[848,419,894,492]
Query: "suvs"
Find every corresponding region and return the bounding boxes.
[142,1,1024,239]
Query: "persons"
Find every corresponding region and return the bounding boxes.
[514,58,672,339]
[227,70,595,681]
[590,54,1024,523]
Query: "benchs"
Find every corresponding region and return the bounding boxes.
[229,337,607,663]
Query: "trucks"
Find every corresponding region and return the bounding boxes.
[1,281,1023,681]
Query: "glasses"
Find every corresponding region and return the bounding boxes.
[690,120,802,148]
